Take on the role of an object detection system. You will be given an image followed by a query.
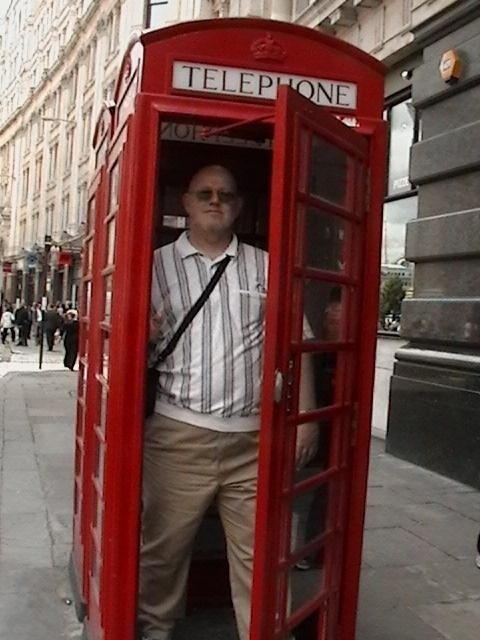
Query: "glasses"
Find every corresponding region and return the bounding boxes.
[187,188,235,204]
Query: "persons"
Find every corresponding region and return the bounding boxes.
[136,162,319,639]
[0,302,80,372]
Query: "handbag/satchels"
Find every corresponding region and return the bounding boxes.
[144,365,160,417]
